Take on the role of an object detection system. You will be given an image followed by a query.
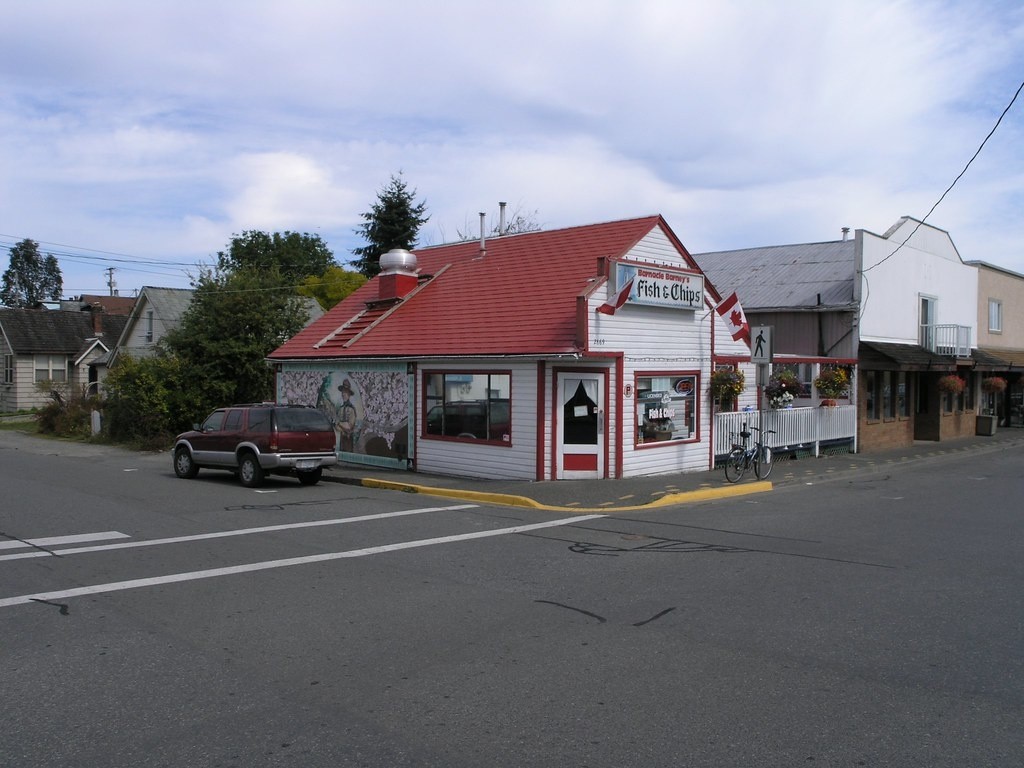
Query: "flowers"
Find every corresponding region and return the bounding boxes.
[764,366,800,409]
[704,368,745,401]
[812,362,850,401]
[980,376,1008,396]
[939,374,965,398]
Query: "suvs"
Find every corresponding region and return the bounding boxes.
[426,398,510,441]
[170,400,338,488]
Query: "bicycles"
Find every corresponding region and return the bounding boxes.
[724,427,776,484]
